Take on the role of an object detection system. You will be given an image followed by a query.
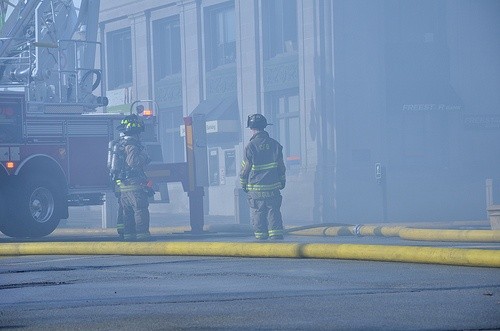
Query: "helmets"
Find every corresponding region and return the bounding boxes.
[116,114,137,130]
[245,114,268,130]
[123,120,142,134]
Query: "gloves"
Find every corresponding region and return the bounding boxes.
[280,182,285,190]
[145,185,155,196]
[242,184,247,193]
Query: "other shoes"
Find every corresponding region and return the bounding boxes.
[119,233,124,240]
[270,235,284,240]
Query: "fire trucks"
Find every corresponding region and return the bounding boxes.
[0,0,209,239]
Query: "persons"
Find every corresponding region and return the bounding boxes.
[117,121,153,240]
[239,113,286,241]
[113,114,138,239]
